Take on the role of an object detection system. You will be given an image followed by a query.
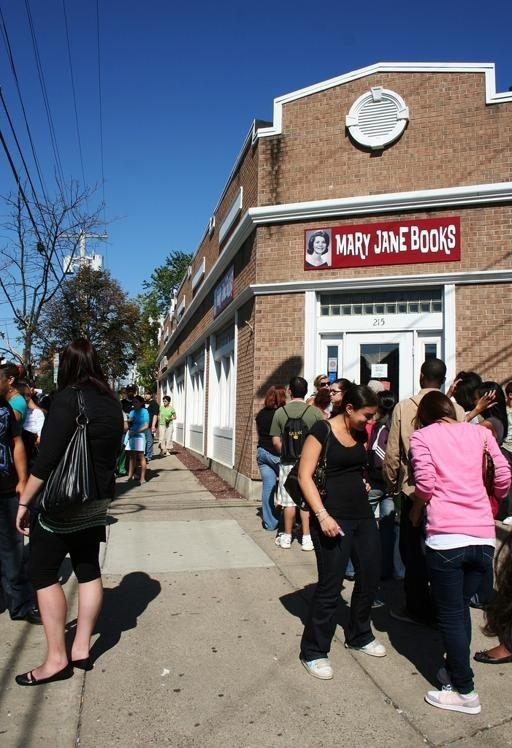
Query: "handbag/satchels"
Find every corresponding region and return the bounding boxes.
[367,420,390,484]
[28,425,96,515]
[283,455,329,512]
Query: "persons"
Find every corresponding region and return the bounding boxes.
[14,339,123,686]
[255,355,512,715]
[2,358,174,625]
[306,230,331,267]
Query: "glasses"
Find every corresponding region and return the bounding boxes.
[332,390,343,395]
[320,382,331,386]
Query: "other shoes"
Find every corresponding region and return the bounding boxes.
[425,684,481,714]
[276,533,292,549]
[301,535,315,550]
[388,606,425,626]
[474,649,512,663]
[437,668,452,684]
[360,639,386,656]
[301,658,333,679]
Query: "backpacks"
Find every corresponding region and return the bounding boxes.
[280,417,309,459]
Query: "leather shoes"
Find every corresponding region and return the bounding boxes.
[16,661,73,686]
[71,657,93,670]
[12,607,43,626]
